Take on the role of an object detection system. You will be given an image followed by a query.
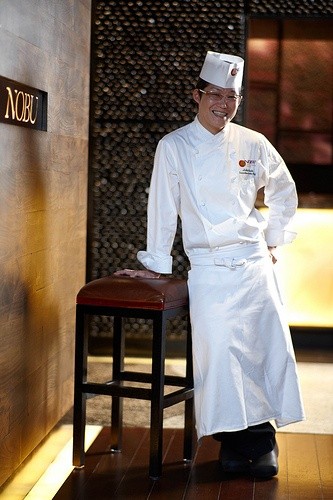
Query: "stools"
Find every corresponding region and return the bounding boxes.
[72,275,196,480]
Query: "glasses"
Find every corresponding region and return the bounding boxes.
[199,88,242,101]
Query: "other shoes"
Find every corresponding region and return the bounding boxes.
[219,440,279,479]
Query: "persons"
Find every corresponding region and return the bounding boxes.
[114,50,303,480]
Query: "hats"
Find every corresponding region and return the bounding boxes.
[198,51,243,88]
